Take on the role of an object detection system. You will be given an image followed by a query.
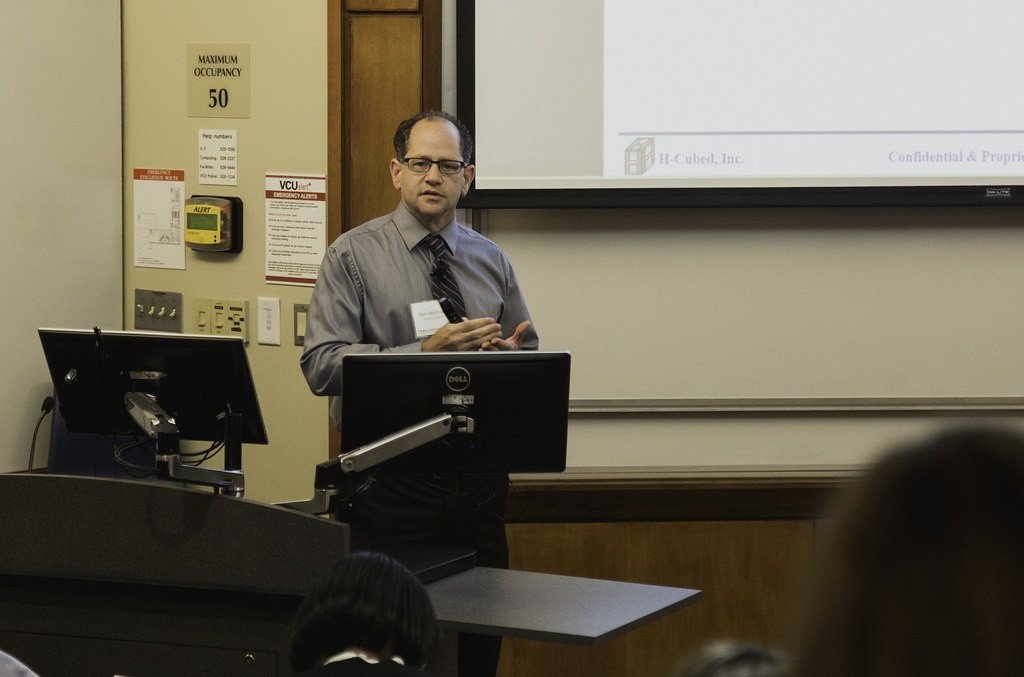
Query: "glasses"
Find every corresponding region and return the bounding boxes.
[401,156,466,175]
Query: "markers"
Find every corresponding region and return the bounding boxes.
[438,297,464,324]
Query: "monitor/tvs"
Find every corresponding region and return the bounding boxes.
[38,328,269,445]
[340,351,571,473]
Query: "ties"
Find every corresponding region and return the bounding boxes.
[424,234,466,324]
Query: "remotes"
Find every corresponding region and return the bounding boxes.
[438,298,463,324]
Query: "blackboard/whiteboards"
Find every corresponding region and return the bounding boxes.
[460,205,1024,417]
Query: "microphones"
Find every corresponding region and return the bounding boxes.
[28,396,54,473]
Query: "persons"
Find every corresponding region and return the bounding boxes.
[301,107,541,674]
[0,422,1024,677]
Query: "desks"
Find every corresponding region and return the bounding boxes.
[425,566,703,677]
